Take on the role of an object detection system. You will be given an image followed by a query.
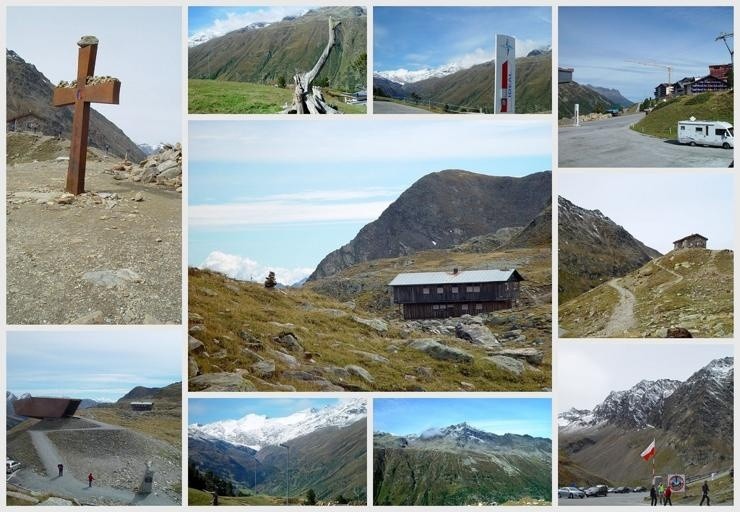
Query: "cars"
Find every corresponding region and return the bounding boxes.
[559,484,646,498]
[6,460,21,473]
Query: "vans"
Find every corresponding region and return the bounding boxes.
[679,120,734,148]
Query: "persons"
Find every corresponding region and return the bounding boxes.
[604,485,609,496]
[664,486,673,506]
[650,485,658,506]
[730,469,734,487]
[700,480,711,506]
[88,473,96,487]
[657,482,665,504]
[58,464,64,476]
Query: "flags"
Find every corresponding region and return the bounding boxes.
[641,440,655,461]
